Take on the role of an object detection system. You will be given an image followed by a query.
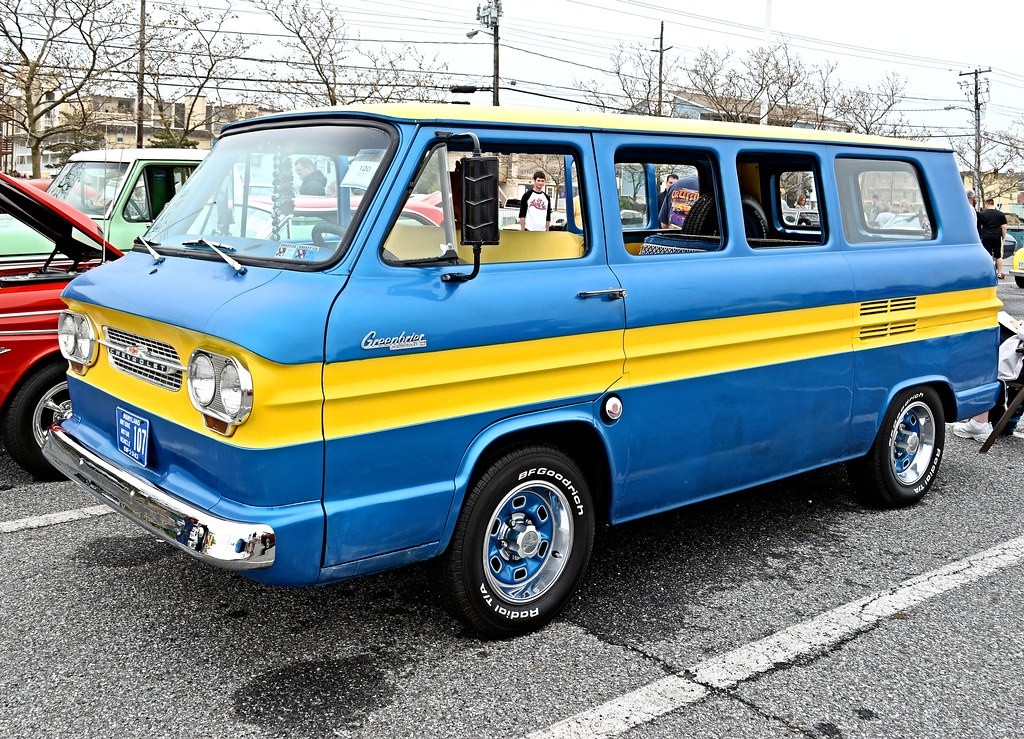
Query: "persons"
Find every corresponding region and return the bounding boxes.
[966,190,977,208]
[518,172,551,232]
[658,178,699,229]
[795,196,812,224]
[295,157,327,196]
[953,320,1024,443]
[978,199,1008,278]
[870,193,910,226]
[658,174,679,214]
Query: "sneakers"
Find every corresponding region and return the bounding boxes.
[953,419,993,442]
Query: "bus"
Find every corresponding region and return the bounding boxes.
[42,103,1008,639]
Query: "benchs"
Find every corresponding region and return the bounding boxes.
[383,225,585,264]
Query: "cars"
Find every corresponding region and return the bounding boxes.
[782,208,819,226]
[226,196,444,242]
[883,213,927,230]
[247,182,299,196]
[1009,247,1024,289]
[0,169,125,482]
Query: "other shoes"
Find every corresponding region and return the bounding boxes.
[997,274,1005,279]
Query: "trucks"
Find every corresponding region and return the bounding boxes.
[0,148,411,265]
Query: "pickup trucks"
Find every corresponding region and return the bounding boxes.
[504,198,521,214]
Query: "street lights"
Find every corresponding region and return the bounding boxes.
[466,29,499,106]
[944,106,980,211]
